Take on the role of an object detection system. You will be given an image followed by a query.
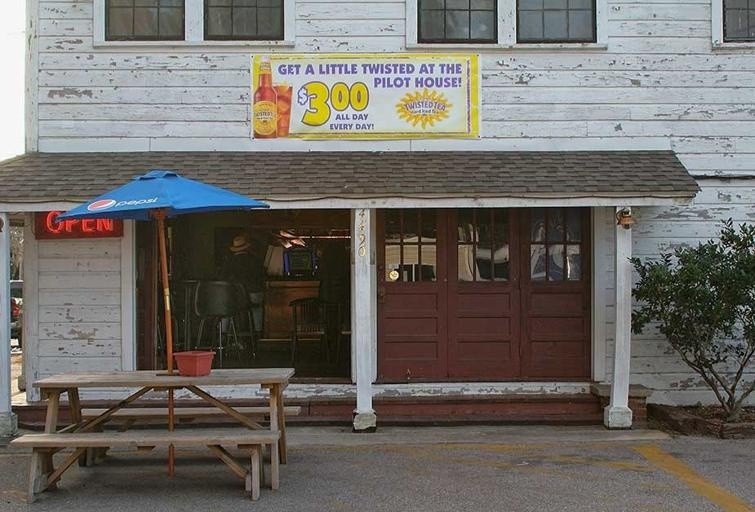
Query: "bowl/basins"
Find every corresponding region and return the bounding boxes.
[172,351,218,375]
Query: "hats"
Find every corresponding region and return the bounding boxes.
[229,235,251,252]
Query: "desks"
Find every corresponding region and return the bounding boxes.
[26,367,298,501]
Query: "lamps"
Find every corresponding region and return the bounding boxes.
[615,208,636,231]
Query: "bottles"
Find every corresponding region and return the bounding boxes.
[253,55,277,139]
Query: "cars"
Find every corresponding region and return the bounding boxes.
[10,277,24,305]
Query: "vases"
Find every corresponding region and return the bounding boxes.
[174,347,215,378]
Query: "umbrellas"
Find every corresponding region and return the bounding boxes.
[53,167,269,475]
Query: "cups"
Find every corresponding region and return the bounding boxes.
[270,81,293,137]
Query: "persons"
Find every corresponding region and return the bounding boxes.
[217,235,266,353]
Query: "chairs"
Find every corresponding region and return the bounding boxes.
[163,274,350,370]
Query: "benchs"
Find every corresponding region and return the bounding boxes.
[69,393,301,465]
[6,430,281,502]
